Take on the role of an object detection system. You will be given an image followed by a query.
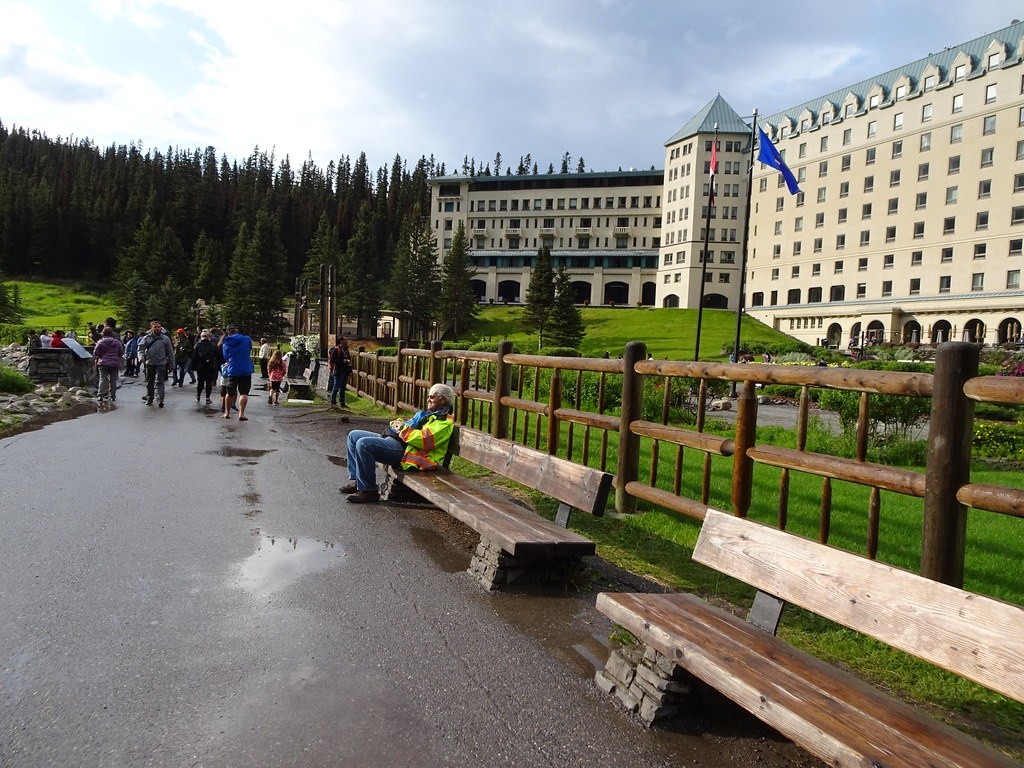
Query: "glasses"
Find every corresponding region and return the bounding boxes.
[428,395,440,399]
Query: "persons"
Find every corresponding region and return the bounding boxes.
[605,351,653,360]
[764,351,771,363]
[819,358,827,366]
[259,338,269,378]
[267,350,286,405]
[328,336,352,409]
[340,383,455,502]
[821,338,829,348]
[864,336,876,347]
[221,324,254,420]
[24,318,240,412]
[730,351,734,364]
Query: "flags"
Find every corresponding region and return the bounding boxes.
[757,125,802,195]
[709,138,717,195]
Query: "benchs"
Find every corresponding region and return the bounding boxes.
[378,424,613,591]
[595,508,1024,768]
[286,368,314,400]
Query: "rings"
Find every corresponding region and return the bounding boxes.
[396,425,398,427]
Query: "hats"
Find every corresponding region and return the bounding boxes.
[200,331,208,338]
[175,328,185,333]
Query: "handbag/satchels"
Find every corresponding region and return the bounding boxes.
[381,409,430,450]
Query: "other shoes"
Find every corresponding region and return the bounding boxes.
[197,396,200,401]
[206,399,212,404]
[97,398,103,401]
[239,416,248,421]
[134,373,139,378]
[274,402,279,404]
[146,401,151,405]
[159,402,164,408]
[221,406,225,413]
[110,398,115,402]
[179,380,183,387]
[189,378,196,384]
[172,379,179,386]
[231,405,239,411]
[341,403,349,408]
[225,416,230,419]
[331,405,335,409]
[268,396,272,404]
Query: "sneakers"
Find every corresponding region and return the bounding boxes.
[346,484,380,503]
[339,480,358,494]
